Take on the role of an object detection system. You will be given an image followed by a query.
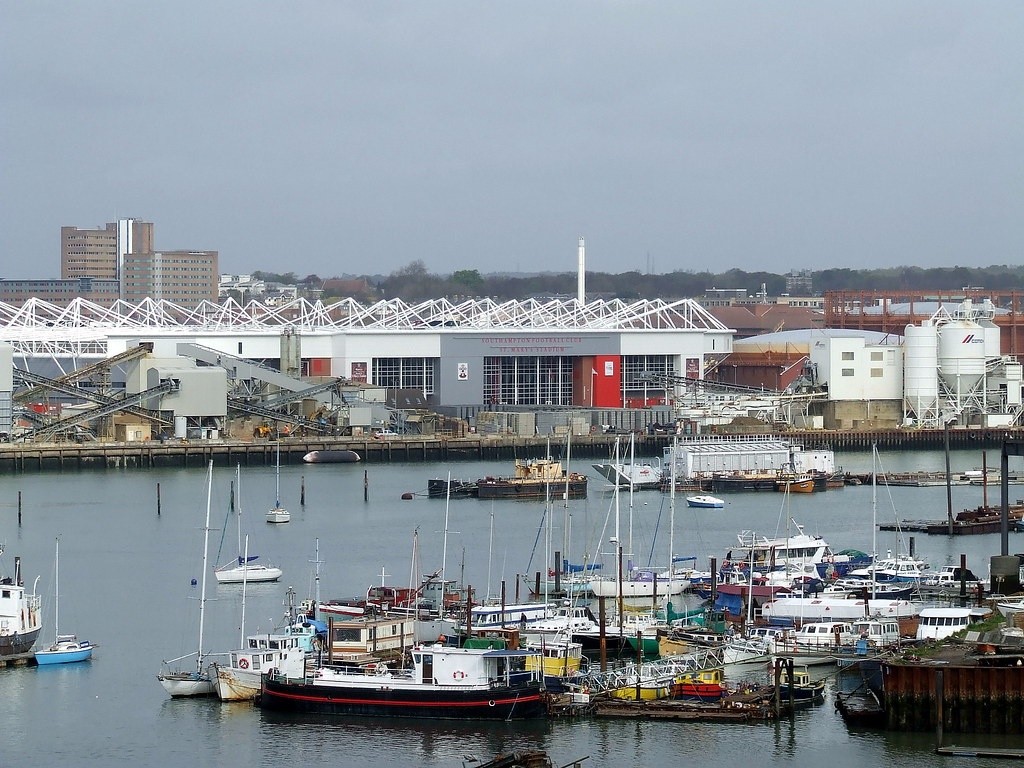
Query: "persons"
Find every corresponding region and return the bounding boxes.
[521,613,527,629]
[857,634,868,656]
[726,551,732,561]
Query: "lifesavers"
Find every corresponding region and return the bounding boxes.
[239,658,250,669]
[453,670,464,682]
[515,484,522,492]
[827,556,834,564]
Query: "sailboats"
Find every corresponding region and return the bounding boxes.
[215,460,283,583]
[265,433,290,523]
[33,537,95,665]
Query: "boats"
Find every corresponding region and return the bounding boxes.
[0,540,45,656]
[156,432,1024,732]
[778,462,816,493]
[594,413,836,488]
[686,495,724,508]
